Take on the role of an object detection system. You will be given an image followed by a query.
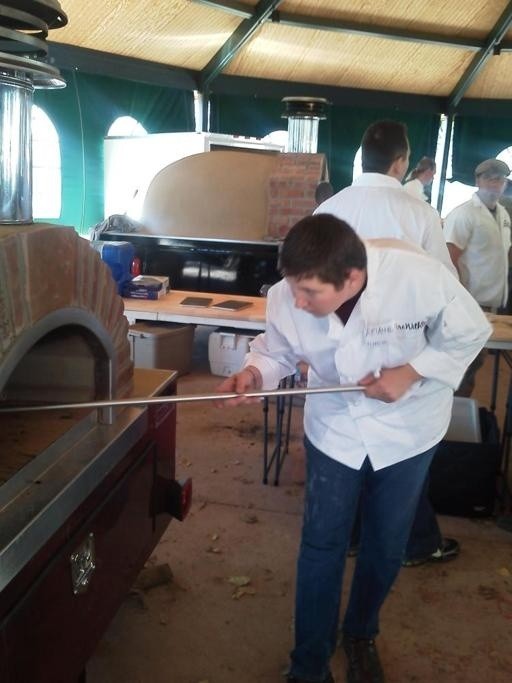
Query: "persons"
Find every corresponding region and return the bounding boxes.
[444,158,511,397]
[402,155,437,202]
[212,212,495,682]
[311,119,462,568]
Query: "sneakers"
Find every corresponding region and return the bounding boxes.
[342,631,384,683]
[402,539,460,567]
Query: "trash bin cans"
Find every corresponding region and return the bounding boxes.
[421,405,502,520]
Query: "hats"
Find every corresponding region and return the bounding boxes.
[475,158,511,176]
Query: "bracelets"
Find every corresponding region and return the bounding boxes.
[244,367,258,391]
